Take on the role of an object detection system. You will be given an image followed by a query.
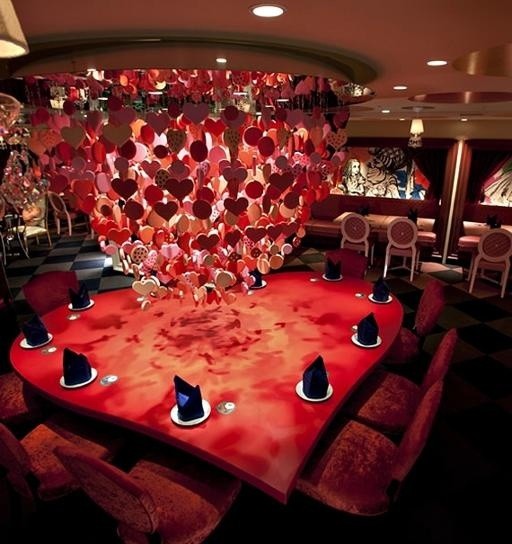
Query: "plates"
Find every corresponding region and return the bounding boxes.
[250,280,267,289]
[295,273,395,403]
[20,299,211,427]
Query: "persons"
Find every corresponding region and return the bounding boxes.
[337,157,366,196]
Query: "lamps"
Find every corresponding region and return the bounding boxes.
[407,116,425,149]
[21,57,353,310]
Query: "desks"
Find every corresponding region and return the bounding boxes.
[7,275,439,504]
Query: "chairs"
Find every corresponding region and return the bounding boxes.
[2,409,146,542]
[340,212,511,299]
[4,180,86,265]
[342,327,457,437]
[293,381,444,521]
[50,441,242,544]
[385,279,445,372]
[22,267,81,322]
